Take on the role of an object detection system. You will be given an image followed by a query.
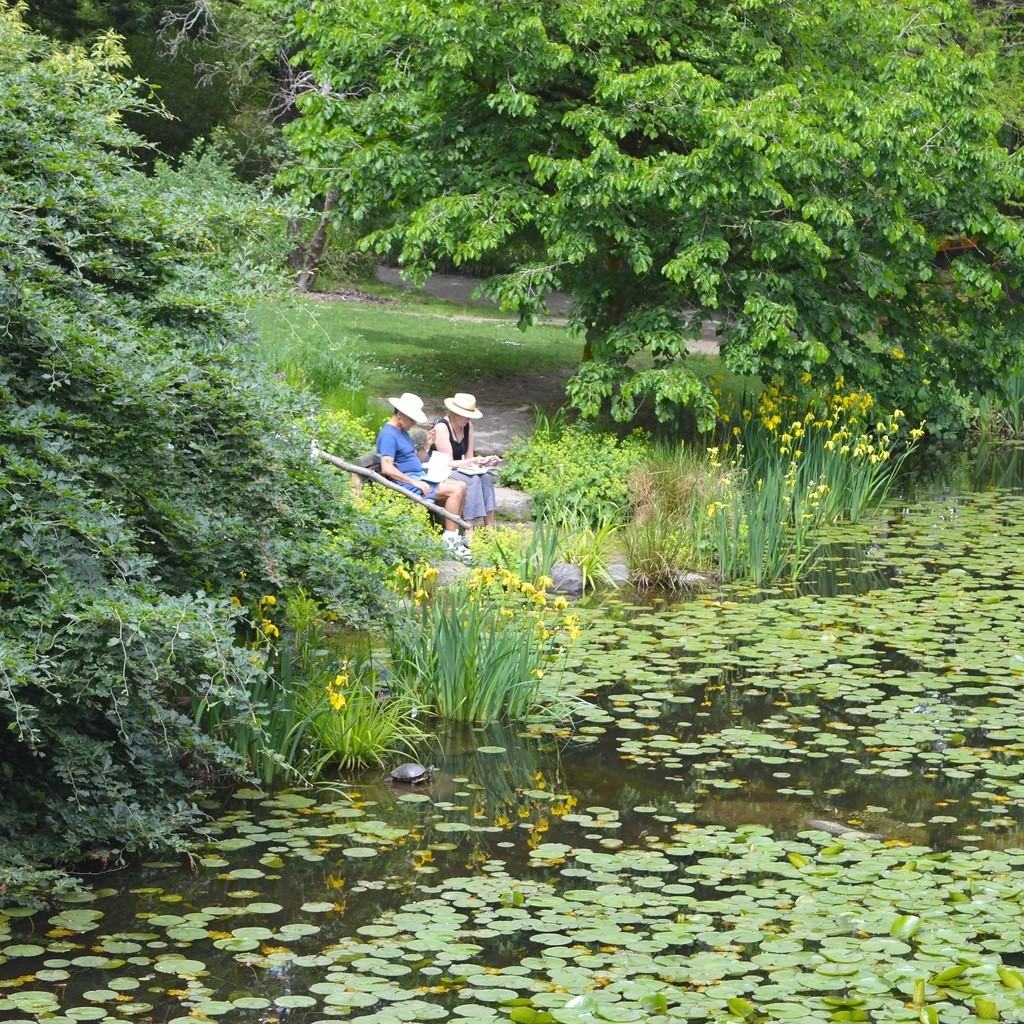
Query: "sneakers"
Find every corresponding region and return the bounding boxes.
[441,534,473,559]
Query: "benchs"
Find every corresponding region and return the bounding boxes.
[353,430,435,509]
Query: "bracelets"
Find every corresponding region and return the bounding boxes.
[422,446,429,452]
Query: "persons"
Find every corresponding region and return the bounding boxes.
[376,392,471,559]
[426,393,496,548]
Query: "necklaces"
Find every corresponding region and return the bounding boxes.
[449,416,459,440]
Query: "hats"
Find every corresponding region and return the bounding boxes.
[388,392,427,423]
[444,392,483,419]
